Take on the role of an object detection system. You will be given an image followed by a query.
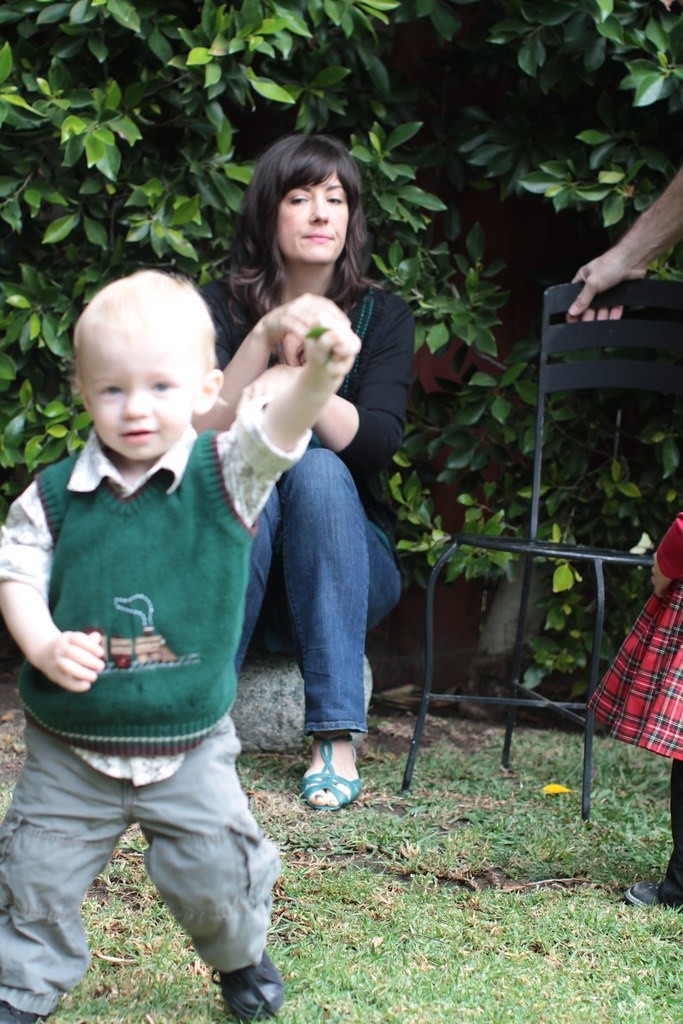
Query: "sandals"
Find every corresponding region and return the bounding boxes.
[301,731,362,811]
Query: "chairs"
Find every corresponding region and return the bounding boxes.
[401,280,683,822]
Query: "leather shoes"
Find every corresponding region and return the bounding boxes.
[625,881,674,911]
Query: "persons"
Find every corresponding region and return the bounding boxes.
[204,136,414,812]
[0,269,362,1023]
[570,163,683,913]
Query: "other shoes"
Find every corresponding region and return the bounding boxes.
[219,946,285,1019]
